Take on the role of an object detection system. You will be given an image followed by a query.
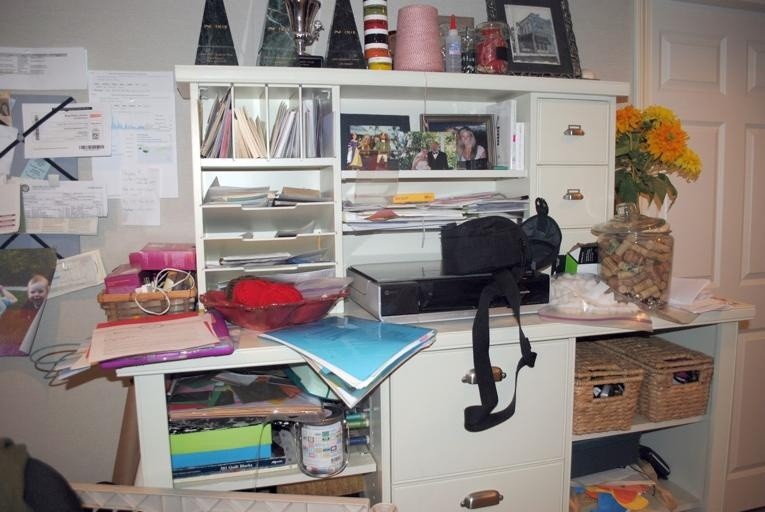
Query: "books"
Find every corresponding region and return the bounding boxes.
[200,86,322,158]
[203,184,331,207]
[256,314,438,411]
[659,297,756,327]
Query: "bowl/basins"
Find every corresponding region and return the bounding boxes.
[198,286,348,332]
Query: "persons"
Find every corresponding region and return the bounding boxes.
[446,126,486,161]
[348,133,409,168]
[427,141,449,170]
[24,275,50,304]
[411,146,431,169]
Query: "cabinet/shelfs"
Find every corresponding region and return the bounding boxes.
[112,297,758,512]
[170,64,632,317]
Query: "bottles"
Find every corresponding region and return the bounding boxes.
[445,14,460,72]
[591,199,673,318]
[471,9,510,73]
[299,408,347,477]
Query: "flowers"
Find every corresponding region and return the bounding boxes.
[615,101,704,213]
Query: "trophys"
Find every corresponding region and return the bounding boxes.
[283,0,322,67]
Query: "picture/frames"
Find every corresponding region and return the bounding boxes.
[419,113,496,170]
[484,0,586,79]
[341,113,411,171]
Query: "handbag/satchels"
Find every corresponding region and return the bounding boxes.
[441,217,524,275]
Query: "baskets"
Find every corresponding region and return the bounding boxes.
[98,275,195,322]
[573,337,714,434]
